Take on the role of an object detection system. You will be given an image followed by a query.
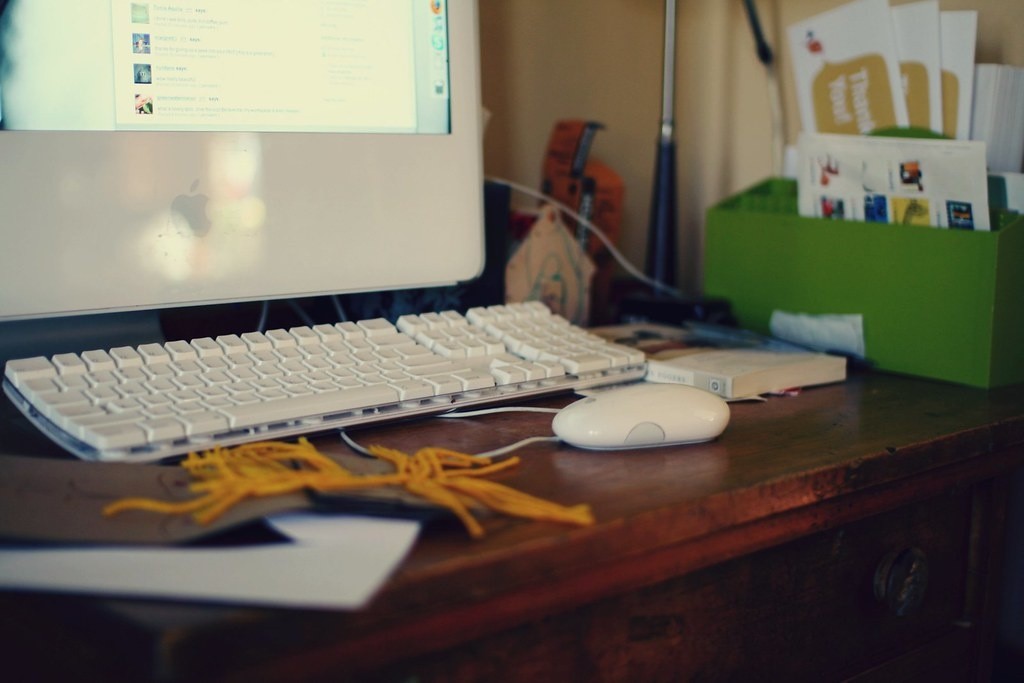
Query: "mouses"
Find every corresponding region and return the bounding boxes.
[552,383,730,451]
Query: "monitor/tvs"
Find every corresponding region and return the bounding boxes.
[0,0,490,361]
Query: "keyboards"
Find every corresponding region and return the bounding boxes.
[0,300,650,466]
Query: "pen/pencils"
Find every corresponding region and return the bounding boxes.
[577,192,594,251]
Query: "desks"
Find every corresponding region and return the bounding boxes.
[0,289,1024,683]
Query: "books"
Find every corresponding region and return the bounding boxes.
[591,324,846,401]
